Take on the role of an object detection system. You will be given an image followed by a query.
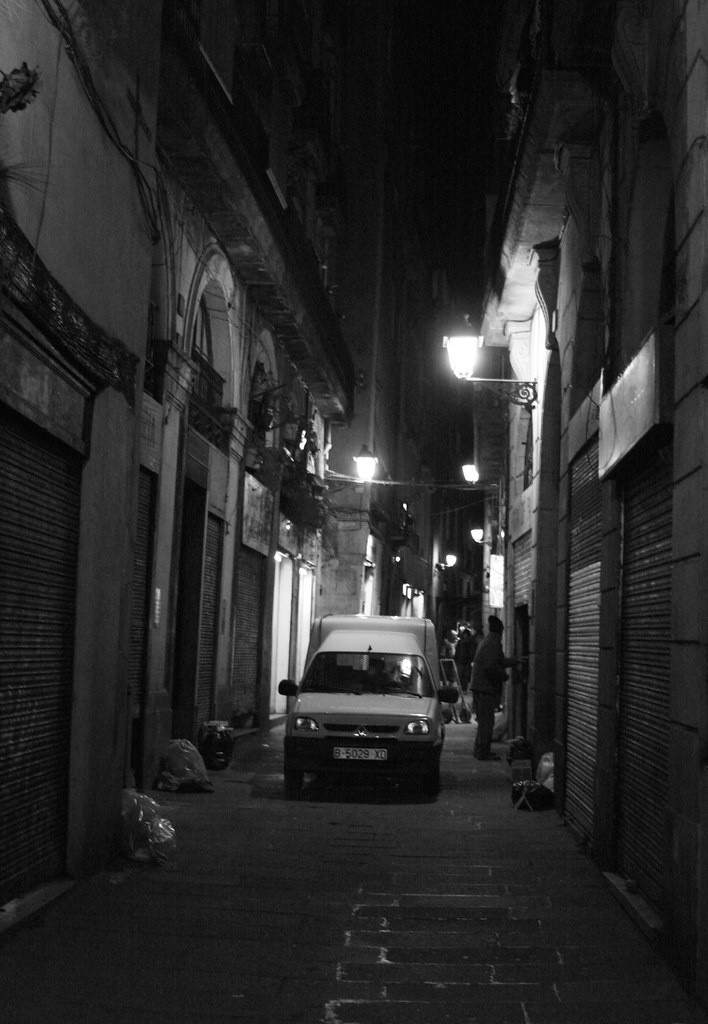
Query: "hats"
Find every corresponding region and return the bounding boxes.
[487,615,504,632]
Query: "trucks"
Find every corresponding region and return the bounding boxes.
[278,614,460,803]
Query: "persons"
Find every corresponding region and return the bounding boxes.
[440,624,484,694]
[469,615,529,762]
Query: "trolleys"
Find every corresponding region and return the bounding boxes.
[438,657,472,725]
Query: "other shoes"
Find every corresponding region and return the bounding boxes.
[474,751,501,760]
